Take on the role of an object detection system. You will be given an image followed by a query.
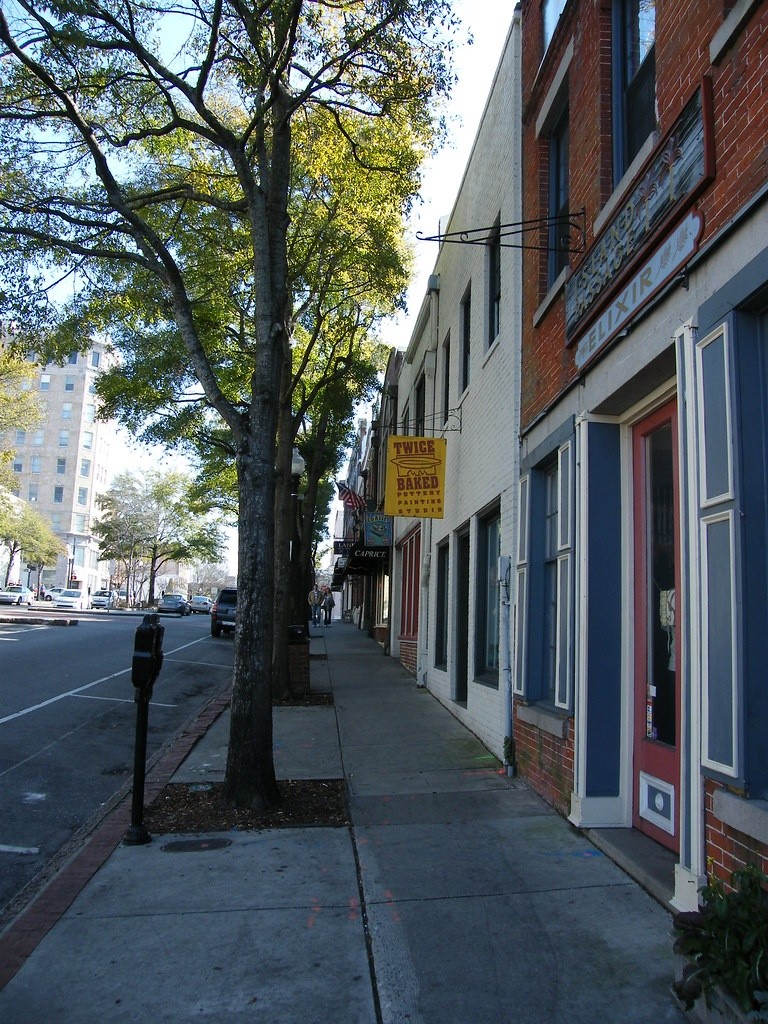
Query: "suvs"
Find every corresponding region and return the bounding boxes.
[211,586,238,636]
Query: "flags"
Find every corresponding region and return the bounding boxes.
[336,482,367,510]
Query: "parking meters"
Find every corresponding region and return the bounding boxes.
[86,587,91,609]
[122,612,165,845]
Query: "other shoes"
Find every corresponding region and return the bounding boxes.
[313,625,316,628]
[317,624,320,628]
[324,625,326,628]
[327,623,331,627]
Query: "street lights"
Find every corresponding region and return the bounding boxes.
[67,554,74,589]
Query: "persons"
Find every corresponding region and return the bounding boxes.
[308,584,324,628]
[321,587,335,628]
[41,585,45,590]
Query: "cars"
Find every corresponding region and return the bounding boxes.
[118,590,132,602]
[44,588,66,601]
[0,586,34,605]
[188,596,212,615]
[90,590,119,609]
[158,594,192,616]
[53,589,92,609]
